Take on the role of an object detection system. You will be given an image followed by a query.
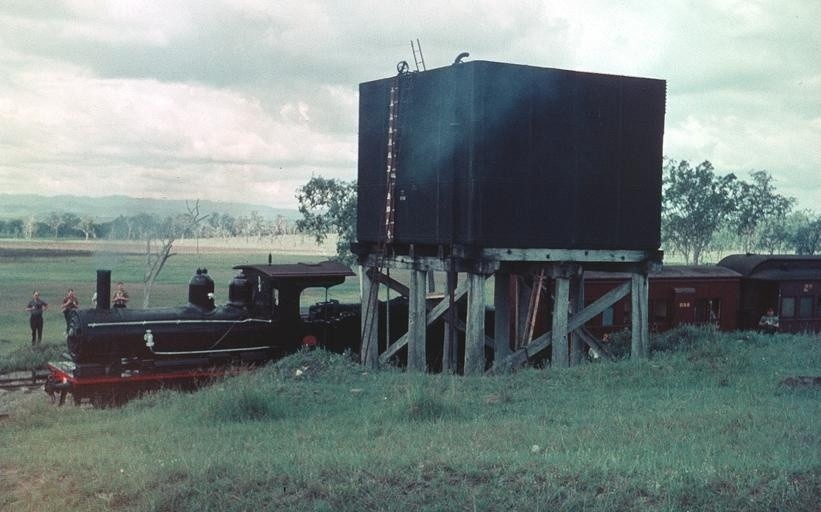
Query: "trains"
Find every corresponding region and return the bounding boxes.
[45,250,821,410]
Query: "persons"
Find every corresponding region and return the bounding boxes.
[757,306,781,337]
[62,288,79,335]
[112,281,130,308]
[24,290,50,347]
